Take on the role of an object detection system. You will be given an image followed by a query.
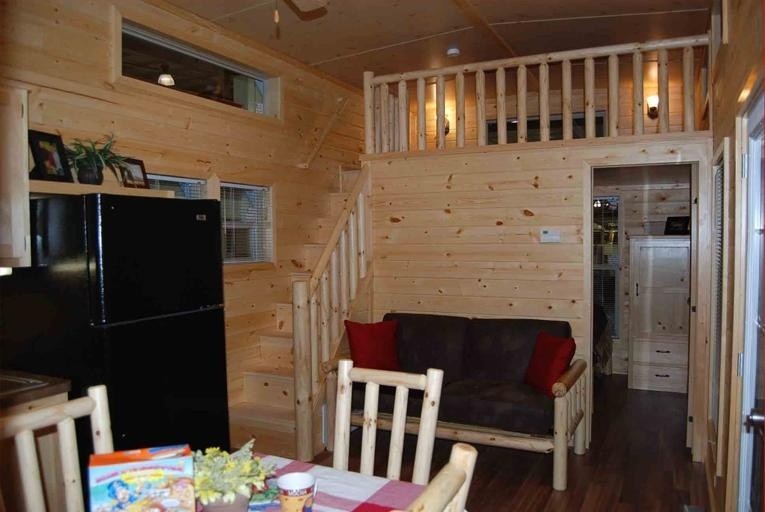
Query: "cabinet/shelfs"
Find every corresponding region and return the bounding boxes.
[628,236,690,395]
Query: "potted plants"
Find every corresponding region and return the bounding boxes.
[61,131,129,185]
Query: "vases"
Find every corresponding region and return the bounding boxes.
[201,493,252,512]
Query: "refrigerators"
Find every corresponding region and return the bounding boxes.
[2,191,231,512]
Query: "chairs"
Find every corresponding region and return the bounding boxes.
[0,385,115,512]
[407,442,480,512]
[333,359,444,486]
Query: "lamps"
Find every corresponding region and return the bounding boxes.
[444,114,449,135]
[273,0,280,24]
[648,89,662,118]
[447,48,458,58]
[157,62,175,86]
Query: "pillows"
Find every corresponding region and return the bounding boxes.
[524,329,576,400]
[342,314,400,371]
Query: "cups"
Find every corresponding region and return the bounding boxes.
[277,472,317,511]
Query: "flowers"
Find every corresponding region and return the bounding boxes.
[193,438,276,504]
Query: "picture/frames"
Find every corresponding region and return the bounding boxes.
[120,158,150,189]
[28,130,74,183]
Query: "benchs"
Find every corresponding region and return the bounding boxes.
[320,310,587,490]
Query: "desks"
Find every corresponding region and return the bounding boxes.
[92,451,428,512]
[0,370,72,512]
